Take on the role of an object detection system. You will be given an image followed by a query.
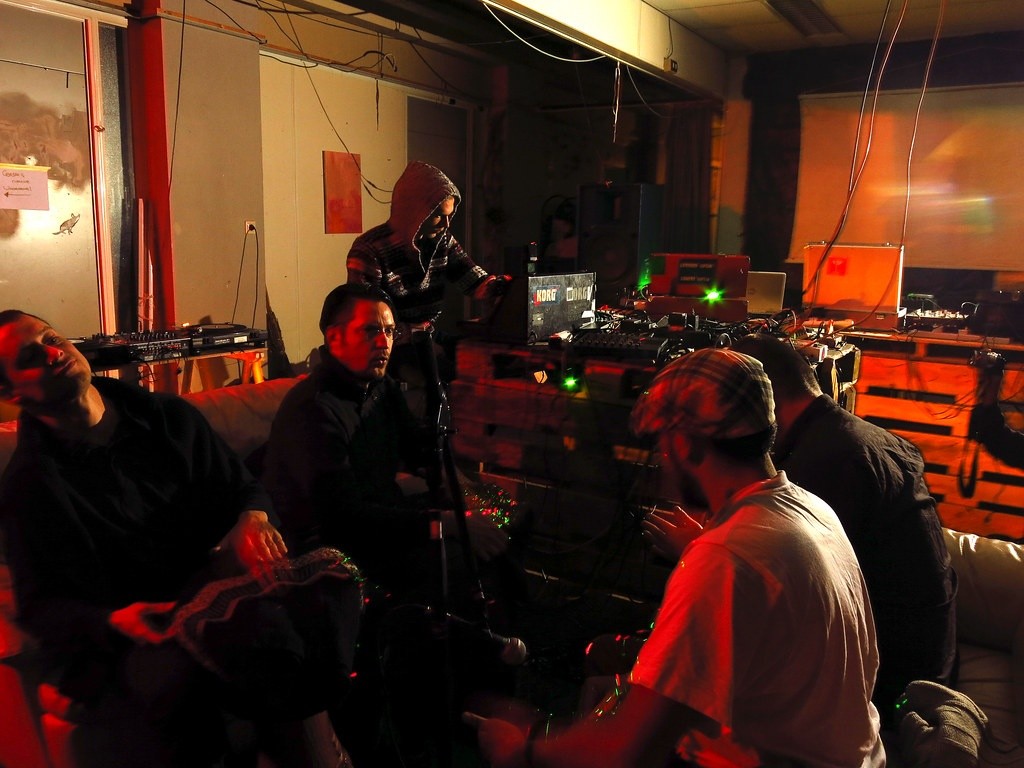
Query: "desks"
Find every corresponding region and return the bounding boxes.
[851,325,1024,547]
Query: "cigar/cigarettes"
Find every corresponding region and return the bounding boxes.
[463,712,486,723]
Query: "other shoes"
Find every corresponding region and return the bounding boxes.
[585,632,648,677]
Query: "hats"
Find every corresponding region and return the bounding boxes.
[626,345,778,440]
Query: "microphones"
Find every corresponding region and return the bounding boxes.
[421,607,527,664]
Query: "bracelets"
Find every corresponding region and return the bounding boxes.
[525,732,537,768]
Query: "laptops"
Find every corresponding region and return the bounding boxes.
[747,271,786,318]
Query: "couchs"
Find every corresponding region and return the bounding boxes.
[841,526,1024,768]
[0,377,298,768]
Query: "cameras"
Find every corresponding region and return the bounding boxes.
[972,350,1006,371]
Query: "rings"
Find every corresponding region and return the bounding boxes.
[658,532,665,537]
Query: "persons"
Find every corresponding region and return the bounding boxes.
[478,348,888,768]
[0,309,363,768]
[263,282,512,670]
[347,158,503,436]
[584,335,956,733]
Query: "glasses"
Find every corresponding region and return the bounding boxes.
[345,322,404,345]
